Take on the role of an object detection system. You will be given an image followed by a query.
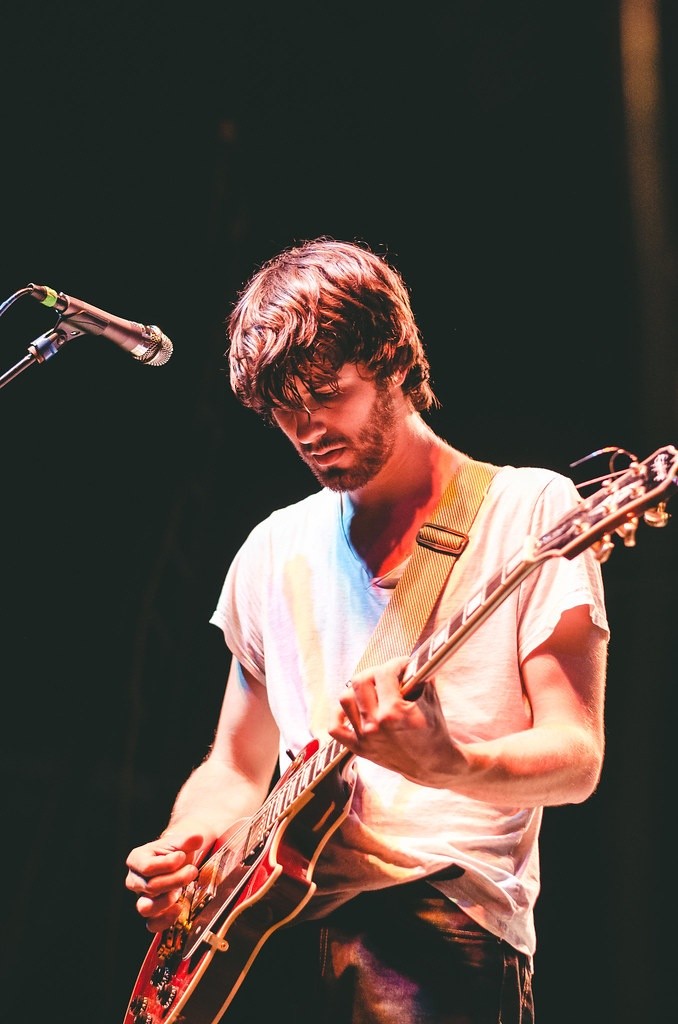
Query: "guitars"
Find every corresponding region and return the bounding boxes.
[111,437,678,1024]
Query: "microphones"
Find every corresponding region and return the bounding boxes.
[27,282,173,366]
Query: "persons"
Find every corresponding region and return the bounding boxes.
[124,238,612,1024]
